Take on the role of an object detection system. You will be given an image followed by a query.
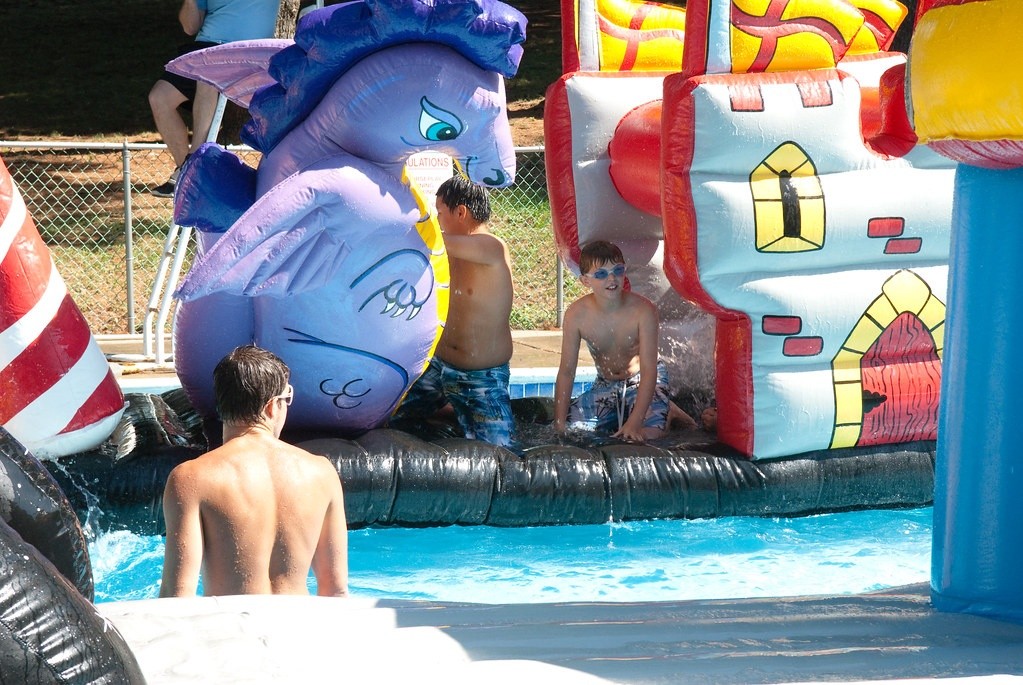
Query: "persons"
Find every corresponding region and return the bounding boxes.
[149,0,283,197]
[158,344,349,598]
[389,175,524,460]
[552,241,697,443]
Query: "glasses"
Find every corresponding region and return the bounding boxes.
[583,264,627,280]
[267,383,294,407]
